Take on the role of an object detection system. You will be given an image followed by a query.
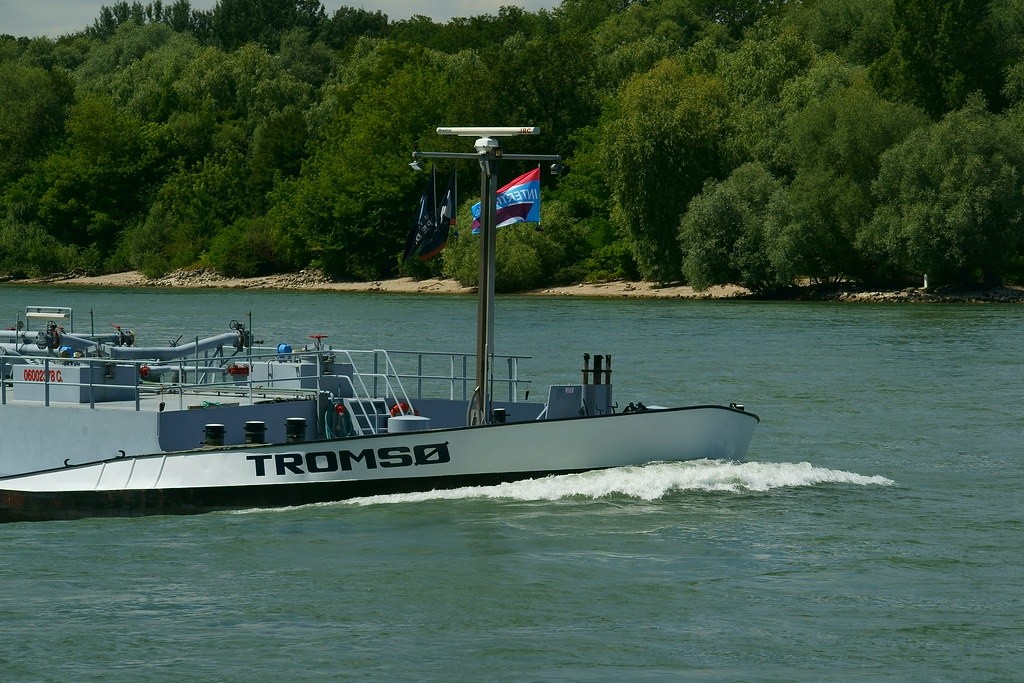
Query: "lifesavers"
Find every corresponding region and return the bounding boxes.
[391,403,419,417]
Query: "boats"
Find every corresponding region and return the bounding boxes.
[0,126,760,524]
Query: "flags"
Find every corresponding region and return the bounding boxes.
[402,160,458,265]
[471,165,540,235]
